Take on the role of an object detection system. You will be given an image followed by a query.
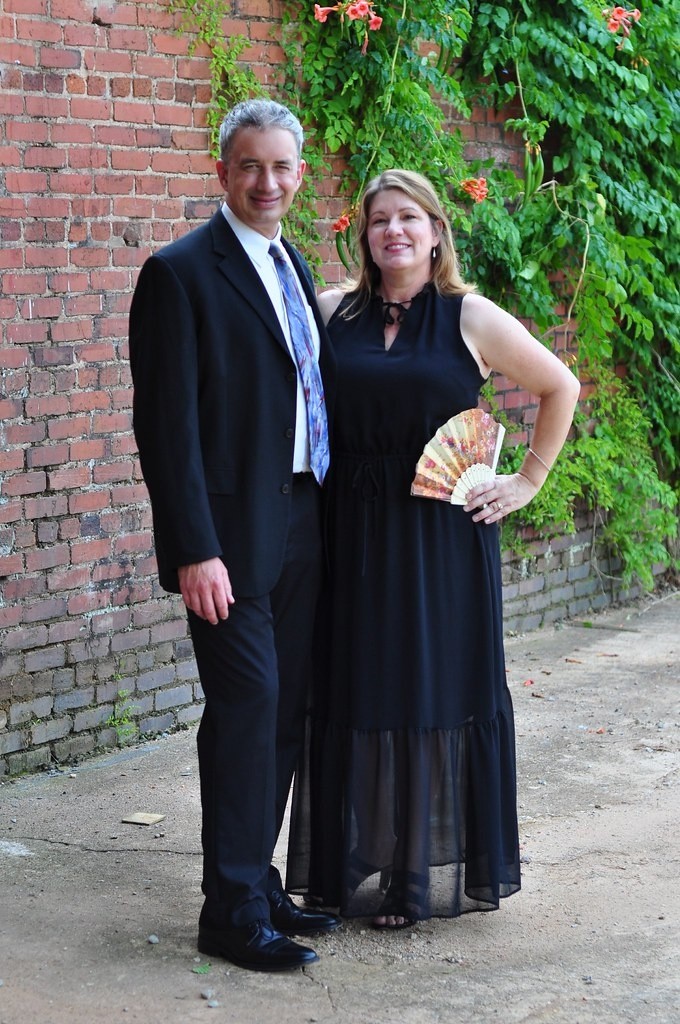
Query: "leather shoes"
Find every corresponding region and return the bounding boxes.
[197,917,318,972]
[267,889,343,935]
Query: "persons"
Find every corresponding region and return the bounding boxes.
[124,98,344,975]
[310,167,582,930]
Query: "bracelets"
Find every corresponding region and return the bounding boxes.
[528,447,551,471]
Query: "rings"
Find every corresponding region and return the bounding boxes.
[495,502,503,509]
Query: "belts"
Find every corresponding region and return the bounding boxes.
[293,473,315,485]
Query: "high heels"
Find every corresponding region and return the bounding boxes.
[371,870,431,929]
[303,845,396,908]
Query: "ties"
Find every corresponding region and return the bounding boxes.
[268,243,330,489]
